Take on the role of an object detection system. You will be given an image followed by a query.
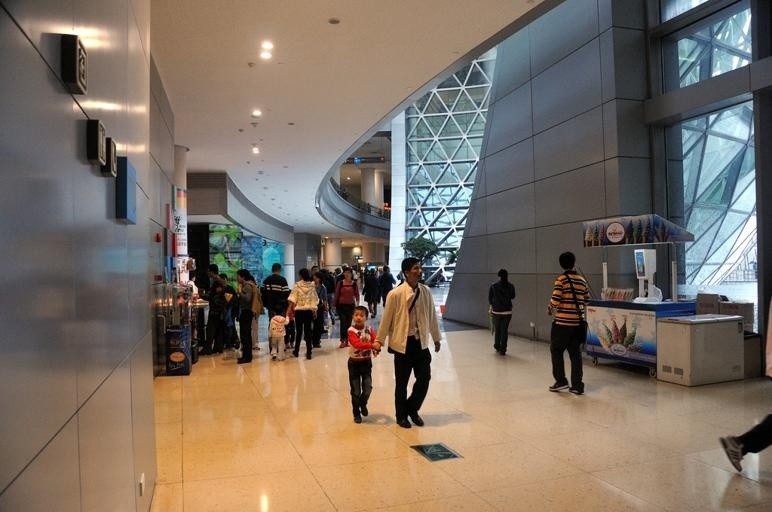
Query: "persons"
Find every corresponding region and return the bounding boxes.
[347,306,381,424]
[718,413,772,473]
[488,267,516,356]
[373,256,443,429]
[197,261,405,365]
[545,251,592,395]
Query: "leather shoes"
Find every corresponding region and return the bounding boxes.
[406,401,424,426]
[199,340,260,355]
[236,356,252,363]
[396,417,411,428]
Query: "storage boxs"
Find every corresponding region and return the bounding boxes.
[718,301,754,335]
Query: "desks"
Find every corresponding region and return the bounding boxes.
[156,301,209,364]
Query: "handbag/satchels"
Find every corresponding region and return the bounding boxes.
[581,321,588,344]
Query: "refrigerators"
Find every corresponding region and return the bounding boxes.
[656,313,745,386]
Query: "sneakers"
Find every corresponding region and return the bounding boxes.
[269,341,311,360]
[354,415,362,423]
[340,343,349,347]
[313,338,321,348]
[361,406,368,416]
[569,382,584,394]
[549,380,569,391]
[719,435,744,472]
[494,344,505,355]
